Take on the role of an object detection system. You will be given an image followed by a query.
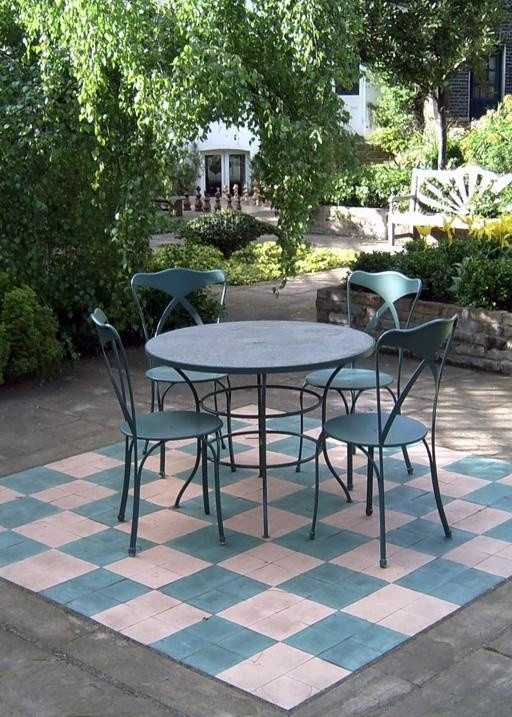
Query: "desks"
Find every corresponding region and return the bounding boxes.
[145,320,377,540]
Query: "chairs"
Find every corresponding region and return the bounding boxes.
[295,270,422,491]
[131,269,232,479]
[310,312,459,566]
[92,308,227,557]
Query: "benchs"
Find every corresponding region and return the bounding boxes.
[387,164,512,247]
